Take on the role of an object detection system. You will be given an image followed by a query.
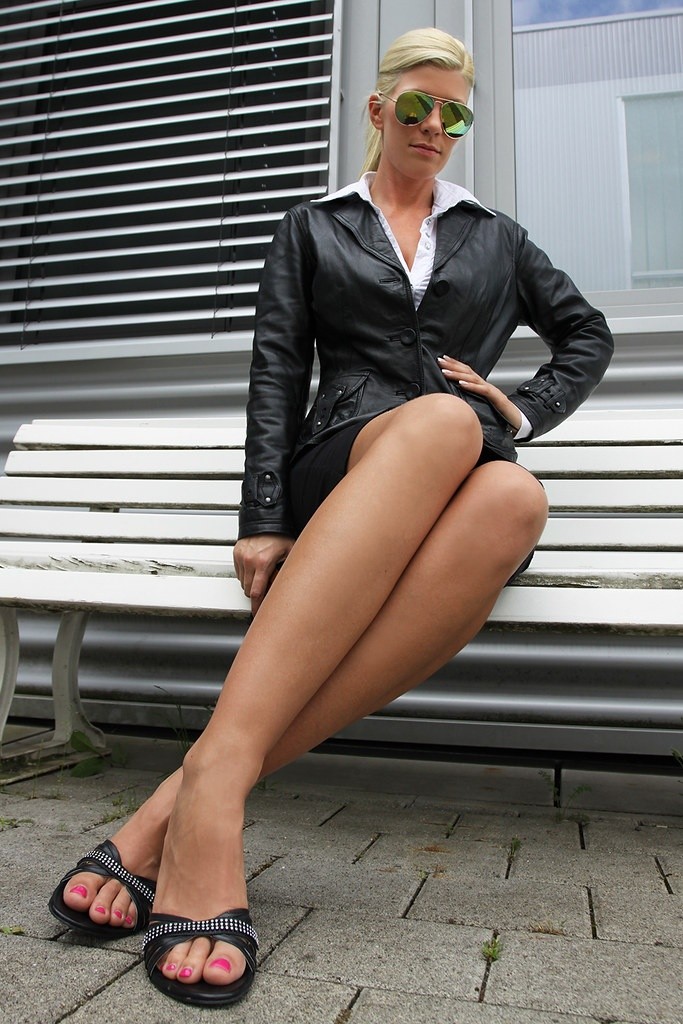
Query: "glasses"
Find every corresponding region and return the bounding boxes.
[379,89,475,139]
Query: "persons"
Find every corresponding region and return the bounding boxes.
[48,27,614,1008]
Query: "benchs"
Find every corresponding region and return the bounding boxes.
[0,408,683,787]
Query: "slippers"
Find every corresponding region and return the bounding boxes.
[50,839,157,937]
[142,907,260,1007]
